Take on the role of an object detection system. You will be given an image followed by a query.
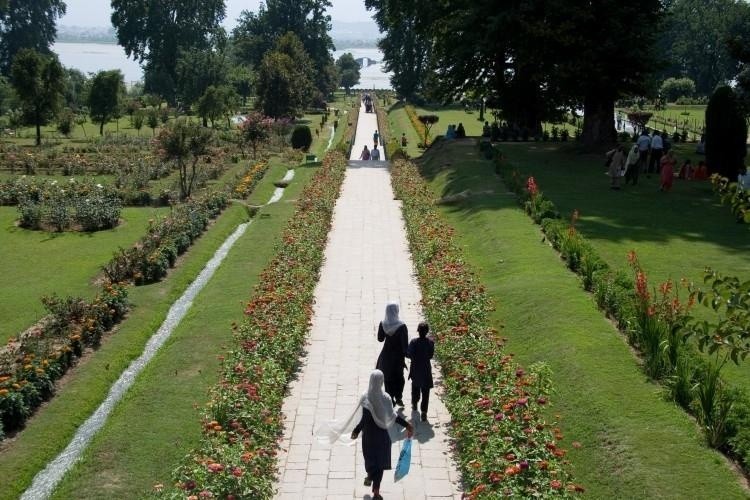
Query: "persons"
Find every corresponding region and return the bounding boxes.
[376,302,409,409]
[358,145,371,160]
[452,125,457,136]
[370,145,380,160]
[457,122,465,136]
[605,127,708,193]
[361,91,373,113]
[446,124,452,135]
[405,320,435,423]
[372,130,379,145]
[401,132,409,147]
[351,368,414,500]
[481,121,491,137]
[735,164,750,191]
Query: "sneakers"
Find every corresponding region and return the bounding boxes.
[392,397,427,421]
[364,476,383,500]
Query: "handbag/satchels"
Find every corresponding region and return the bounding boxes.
[394,438,412,482]
[605,158,612,168]
